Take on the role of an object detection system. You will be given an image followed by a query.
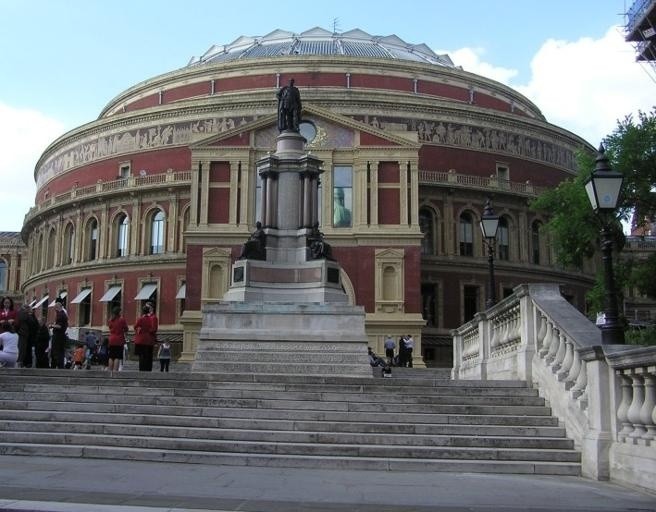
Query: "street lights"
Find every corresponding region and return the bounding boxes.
[478,197,501,310]
[582,141,626,345]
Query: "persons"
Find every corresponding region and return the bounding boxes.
[236,220,268,261]
[368,333,415,378]
[275,77,304,134]
[307,221,333,260]
[2,295,173,372]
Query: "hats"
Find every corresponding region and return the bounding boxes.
[112,306,122,314]
